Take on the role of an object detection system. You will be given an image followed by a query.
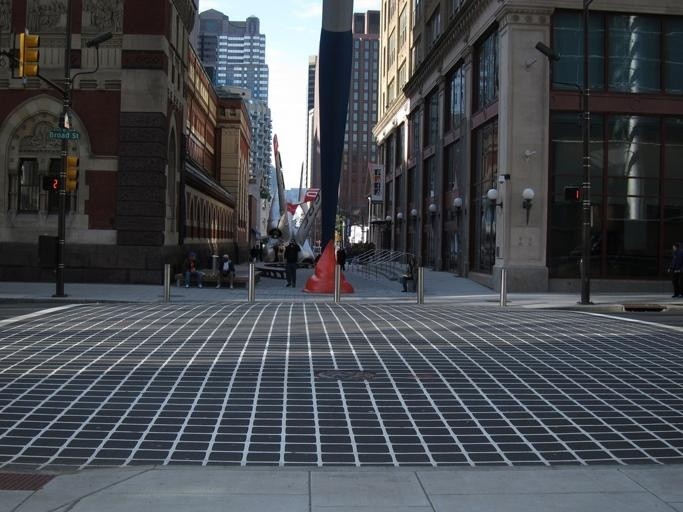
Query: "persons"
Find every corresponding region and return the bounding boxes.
[215,254,235,289]
[249,246,256,262]
[282,239,301,289]
[667,242,682,298]
[182,251,204,288]
[339,249,348,271]
[401,255,418,292]
[255,245,260,260]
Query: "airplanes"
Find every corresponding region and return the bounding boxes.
[261,133,322,268]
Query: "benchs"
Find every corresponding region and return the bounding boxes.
[254,261,309,279]
[175,270,260,289]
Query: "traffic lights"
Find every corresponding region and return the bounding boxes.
[12,32,40,79]
[562,184,583,203]
[43,155,80,194]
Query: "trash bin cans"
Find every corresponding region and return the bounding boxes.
[37,235,59,270]
